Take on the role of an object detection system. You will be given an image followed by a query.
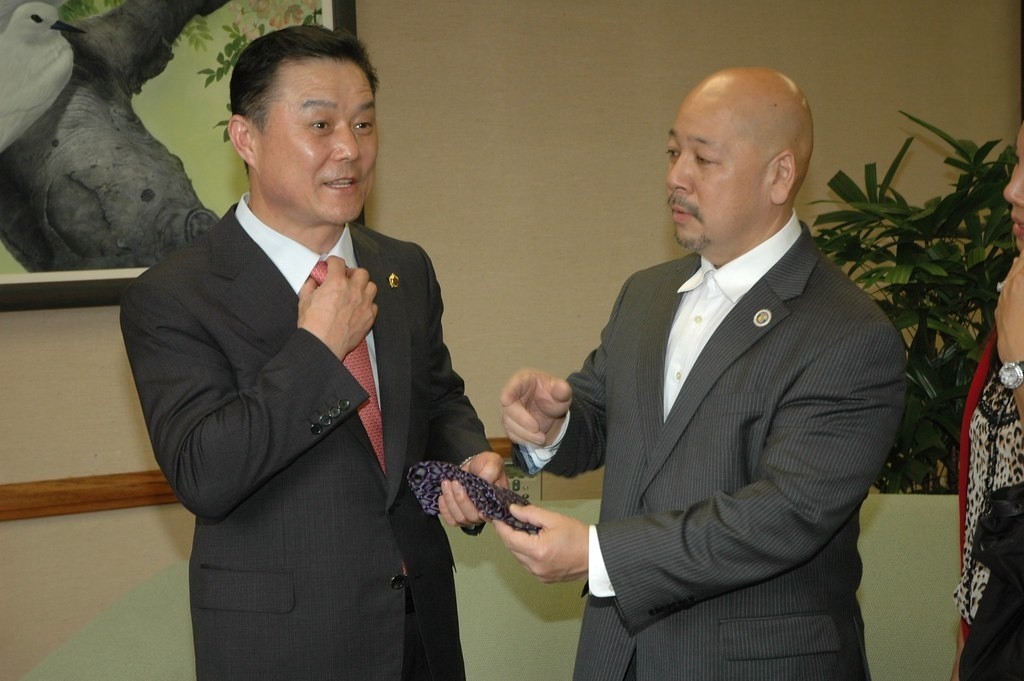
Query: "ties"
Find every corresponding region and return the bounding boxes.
[310,260,408,579]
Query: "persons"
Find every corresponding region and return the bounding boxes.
[117,25,510,681]
[491,67,912,681]
[956,117,1024,679]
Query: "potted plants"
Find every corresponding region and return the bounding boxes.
[803,110,1020,681]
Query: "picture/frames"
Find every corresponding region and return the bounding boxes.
[0,0,363,313]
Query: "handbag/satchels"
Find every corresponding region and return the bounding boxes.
[958,485,1023,679]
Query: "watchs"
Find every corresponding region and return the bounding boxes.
[995,361,1024,391]
